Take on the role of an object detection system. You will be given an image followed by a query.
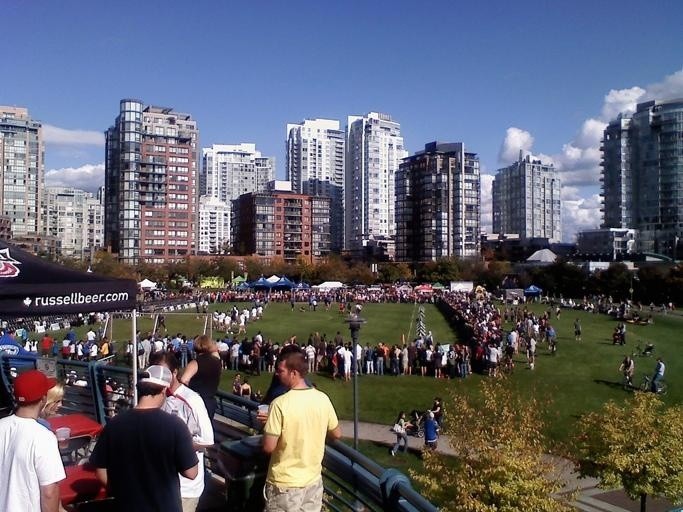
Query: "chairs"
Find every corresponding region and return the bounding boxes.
[0,357,104,465]
[58,465,116,512]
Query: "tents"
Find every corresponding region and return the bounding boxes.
[0,237,138,408]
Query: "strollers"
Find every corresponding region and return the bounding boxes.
[405,408,427,438]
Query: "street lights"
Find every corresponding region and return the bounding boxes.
[629,271,639,308]
[341,305,369,512]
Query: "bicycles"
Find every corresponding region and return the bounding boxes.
[639,375,668,395]
[624,371,632,391]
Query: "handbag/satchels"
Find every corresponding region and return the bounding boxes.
[391,423,405,433]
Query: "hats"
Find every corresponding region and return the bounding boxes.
[14,370,57,403]
[138,365,172,388]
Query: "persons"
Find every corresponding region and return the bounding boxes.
[89,365,198,512]
[151,346,215,512]
[263,349,341,511]
[0,278,557,417]
[391,397,445,458]
[651,357,665,393]
[643,341,655,355]
[618,355,635,385]
[0,370,67,512]
[556,293,676,346]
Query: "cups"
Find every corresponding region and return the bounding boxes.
[55,427,71,450]
[258,403,269,416]
